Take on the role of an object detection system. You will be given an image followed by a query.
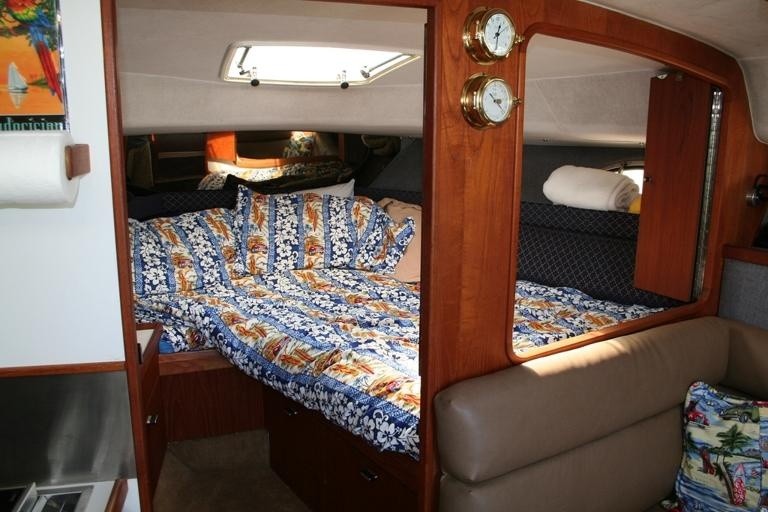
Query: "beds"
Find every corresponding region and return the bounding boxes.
[156,259,675,462]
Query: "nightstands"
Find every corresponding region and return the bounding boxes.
[135,322,166,502]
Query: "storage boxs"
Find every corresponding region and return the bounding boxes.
[265,378,427,512]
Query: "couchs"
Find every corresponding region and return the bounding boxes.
[432,315,768,512]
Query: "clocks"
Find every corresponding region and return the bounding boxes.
[459,71,522,131]
[458,6,524,66]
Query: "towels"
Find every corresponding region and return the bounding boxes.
[542,165,639,212]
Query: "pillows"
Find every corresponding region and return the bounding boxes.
[128,206,248,298]
[292,179,357,196]
[378,197,421,284]
[234,185,416,276]
[282,130,314,168]
[674,381,768,512]
